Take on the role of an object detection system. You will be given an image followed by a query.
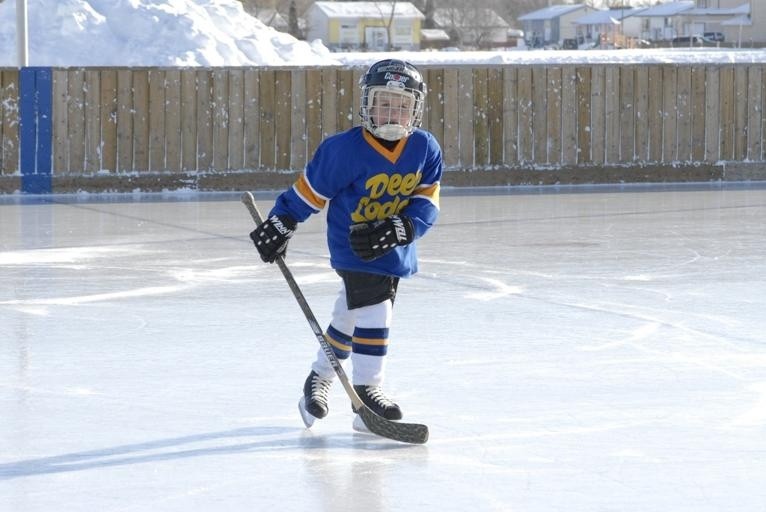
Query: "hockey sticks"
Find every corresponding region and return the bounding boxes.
[242,191,429,445]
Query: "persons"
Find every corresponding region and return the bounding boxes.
[248,57,444,422]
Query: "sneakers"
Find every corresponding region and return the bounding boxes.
[303,366,333,419]
[351,381,402,421]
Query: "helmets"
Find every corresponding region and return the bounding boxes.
[358,58,427,142]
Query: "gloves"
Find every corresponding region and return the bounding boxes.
[249,212,298,264]
[349,214,415,262]
